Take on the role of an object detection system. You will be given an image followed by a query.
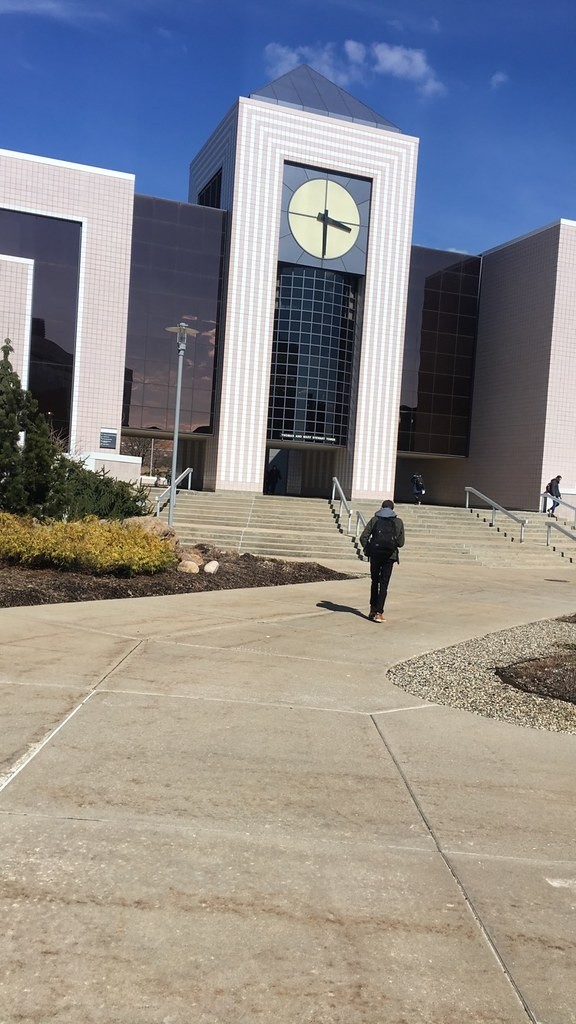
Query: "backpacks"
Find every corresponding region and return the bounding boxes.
[546,481,551,494]
[415,478,424,491]
[369,516,396,560]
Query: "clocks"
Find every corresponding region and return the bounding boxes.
[277,157,375,277]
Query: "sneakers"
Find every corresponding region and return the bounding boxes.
[375,613,386,622]
[368,609,376,620]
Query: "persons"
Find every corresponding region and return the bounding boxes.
[263,465,282,494]
[547,475,562,517]
[360,500,405,623]
[410,471,425,505]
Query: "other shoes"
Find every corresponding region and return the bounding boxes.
[551,514,555,517]
[547,509,551,513]
